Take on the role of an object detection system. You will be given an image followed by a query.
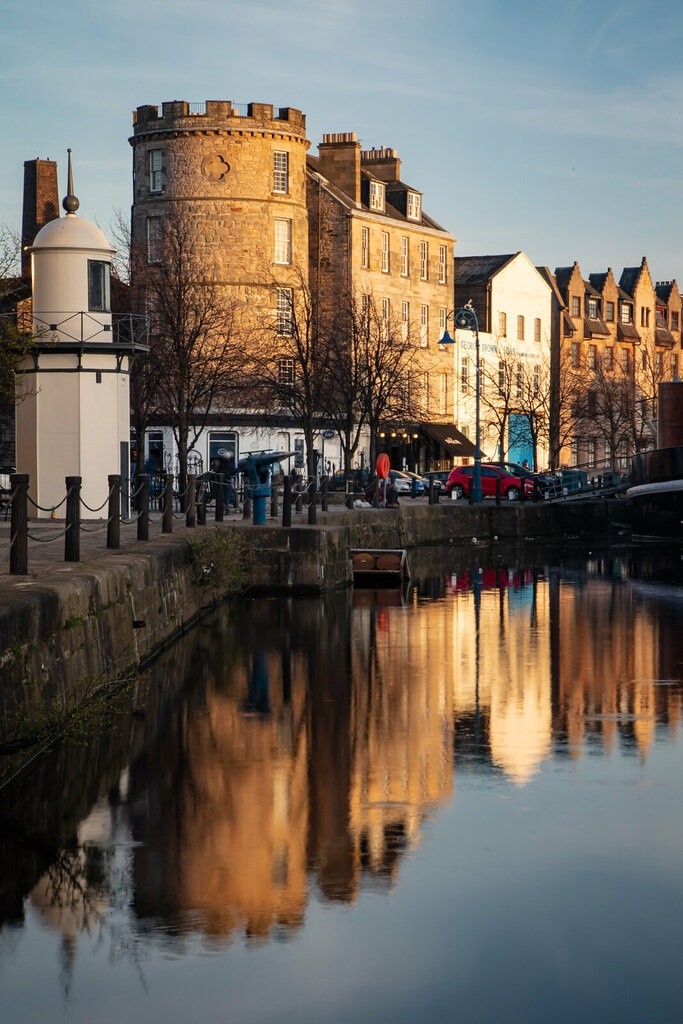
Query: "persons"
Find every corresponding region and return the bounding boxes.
[218,452,238,516]
[131,451,138,483]
[524,463,530,471]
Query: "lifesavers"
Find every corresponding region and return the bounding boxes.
[376,453,391,480]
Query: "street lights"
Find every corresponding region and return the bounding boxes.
[437,307,484,504]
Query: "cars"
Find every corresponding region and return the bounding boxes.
[446,463,541,504]
[330,459,457,498]
[482,460,538,487]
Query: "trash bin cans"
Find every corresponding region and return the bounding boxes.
[434,485,441,503]
